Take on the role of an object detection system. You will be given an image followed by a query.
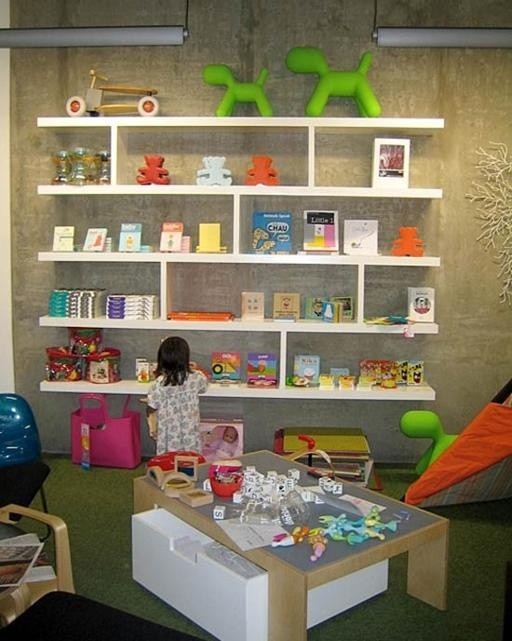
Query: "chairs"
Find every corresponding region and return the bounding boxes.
[1,394,53,544]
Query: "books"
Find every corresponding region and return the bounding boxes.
[239,286,436,326]
[52,221,228,255]
[297,250,339,256]
[0,532,57,583]
[0,542,45,587]
[210,351,424,390]
[251,211,293,255]
[302,210,340,252]
[343,220,379,257]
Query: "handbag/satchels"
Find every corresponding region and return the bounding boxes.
[70,393,142,470]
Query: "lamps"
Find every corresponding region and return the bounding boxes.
[0,0,191,49]
[367,1,512,50]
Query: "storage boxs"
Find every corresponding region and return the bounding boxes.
[129,507,388,641]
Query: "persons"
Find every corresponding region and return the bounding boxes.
[204,427,240,462]
[145,336,209,458]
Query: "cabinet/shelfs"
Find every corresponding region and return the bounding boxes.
[32,118,447,399]
